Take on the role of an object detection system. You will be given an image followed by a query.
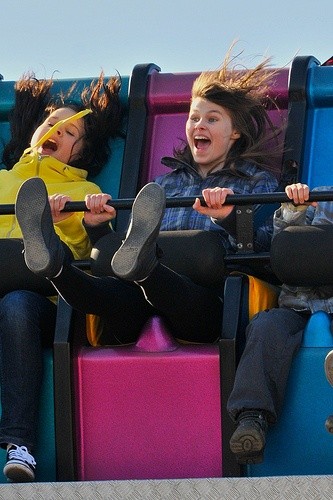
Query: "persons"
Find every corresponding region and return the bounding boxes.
[226,183,333,465]
[0,67,130,484]
[16,45,282,344]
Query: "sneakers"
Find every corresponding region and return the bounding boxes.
[2,442,39,483]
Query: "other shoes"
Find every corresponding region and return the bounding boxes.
[229,407,269,468]
[14,175,65,279]
[110,181,168,280]
